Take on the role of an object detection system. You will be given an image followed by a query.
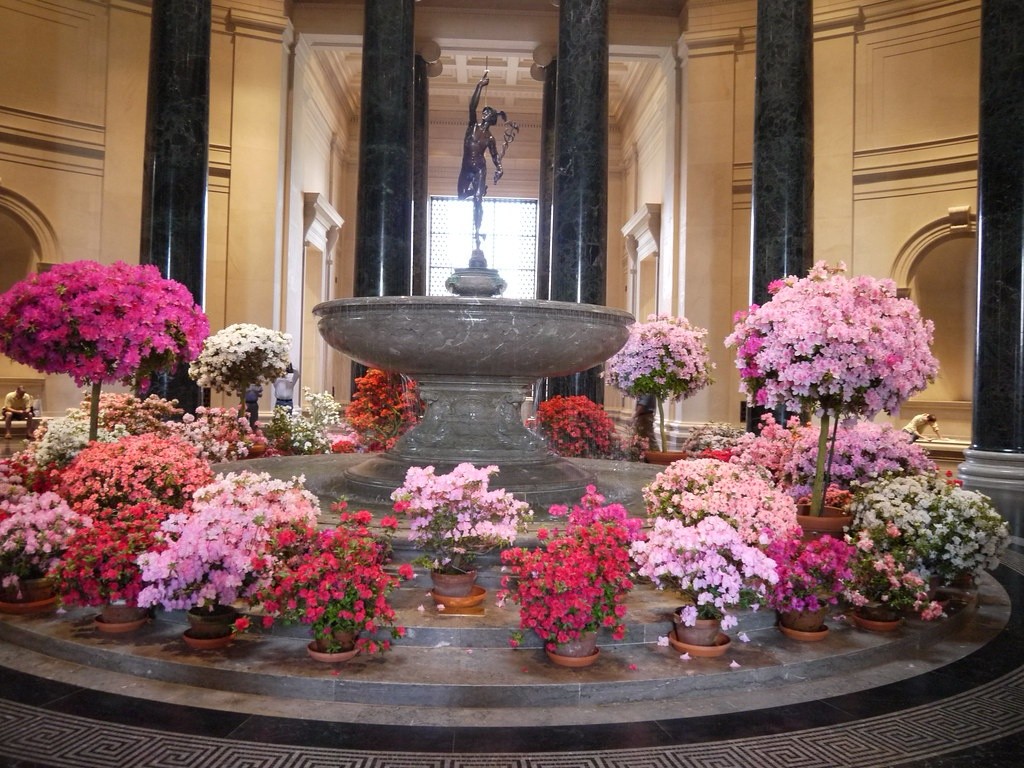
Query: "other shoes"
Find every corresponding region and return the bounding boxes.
[26,435,35,440]
[5,433,13,439]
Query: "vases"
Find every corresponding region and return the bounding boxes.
[850,602,906,633]
[306,622,359,662]
[777,602,831,641]
[93,602,151,633]
[668,606,732,656]
[544,621,600,666]
[792,504,852,559]
[0,577,59,614]
[643,451,688,467]
[178,603,236,652]
[429,565,488,607]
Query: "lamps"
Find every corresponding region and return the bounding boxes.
[419,40,443,78]
[530,45,552,82]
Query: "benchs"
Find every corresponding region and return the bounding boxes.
[0,398,51,436]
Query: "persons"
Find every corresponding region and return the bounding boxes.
[2,385,34,438]
[236,383,263,429]
[457,71,504,249]
[270,369,299,415]
[902,413,942,442]
[632,391,660,452]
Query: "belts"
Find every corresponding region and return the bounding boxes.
[276,398,293,402]
[638,410,654,415]
[245,401,257,404]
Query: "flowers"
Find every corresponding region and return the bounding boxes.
[0,259,1009,658]
